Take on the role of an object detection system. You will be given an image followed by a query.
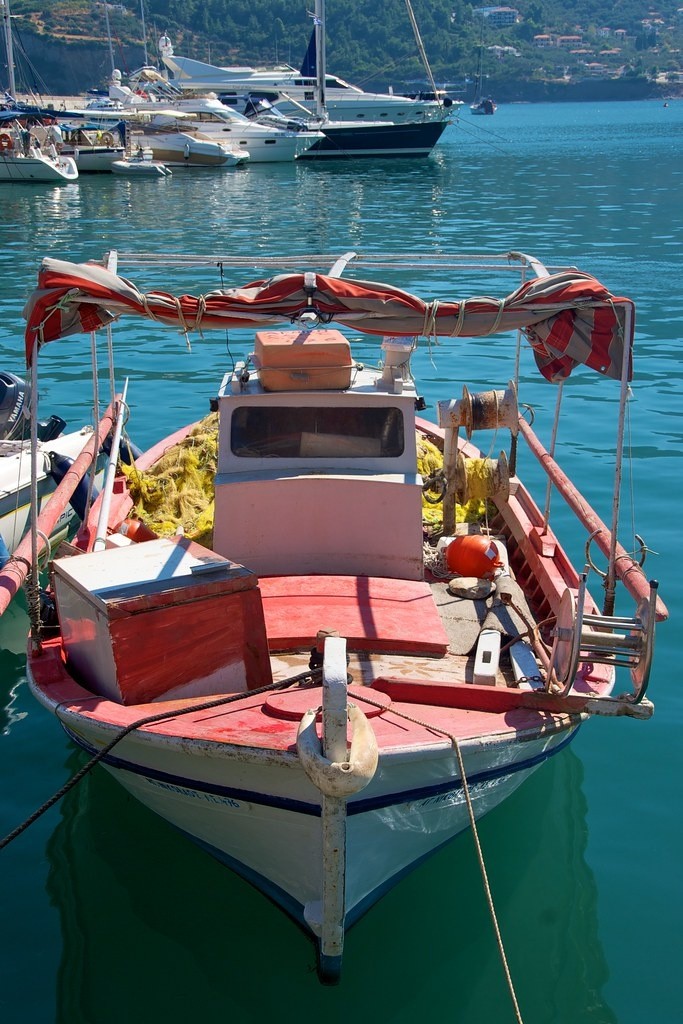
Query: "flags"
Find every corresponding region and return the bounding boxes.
[5,93,14,102]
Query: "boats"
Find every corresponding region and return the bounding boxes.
[0,249,669,985]
[0,0,460,181]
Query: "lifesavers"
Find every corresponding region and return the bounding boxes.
[100,132,114,145]
[0,133,12,151]
[113,518,157,542]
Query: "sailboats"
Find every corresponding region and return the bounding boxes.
[470,6,498,115]
